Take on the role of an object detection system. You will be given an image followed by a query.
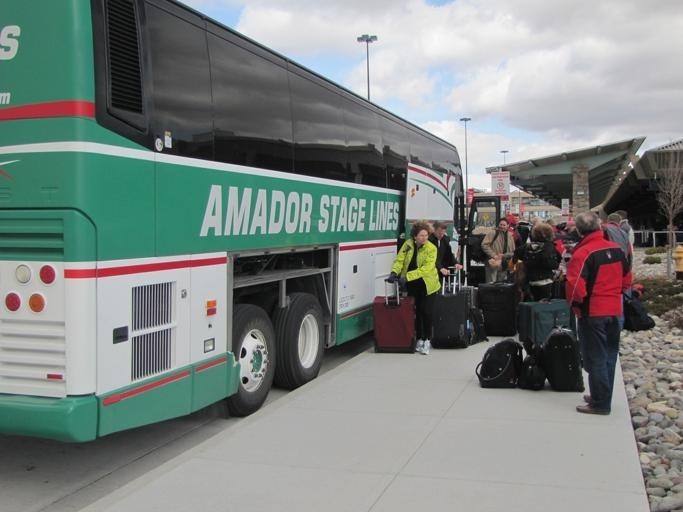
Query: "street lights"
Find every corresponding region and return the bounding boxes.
[500,151,510,165]
[459,115,472,187]
[357,34,378,103]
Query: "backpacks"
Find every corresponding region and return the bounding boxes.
[524,241,556,280]
[472,228,499,263]
[542,324,586,392]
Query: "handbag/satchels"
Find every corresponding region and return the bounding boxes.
[475,338,524,389]
[618,289,656,333]
[518,342,547,391]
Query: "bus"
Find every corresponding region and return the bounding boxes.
[0,1,502,450]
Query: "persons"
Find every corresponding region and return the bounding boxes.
[385,220,442,355]
[428,209,636,303]
[565,210,632,415]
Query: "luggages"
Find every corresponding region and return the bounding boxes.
[371,278,419,354]
[478,281,519,338]
[429,265,480,350]
[518,298,571,345]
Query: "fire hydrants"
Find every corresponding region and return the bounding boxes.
[670,243,683,280]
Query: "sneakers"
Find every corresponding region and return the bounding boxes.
[412,336,433,356]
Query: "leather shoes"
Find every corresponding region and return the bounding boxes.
[574,393,612,416]
